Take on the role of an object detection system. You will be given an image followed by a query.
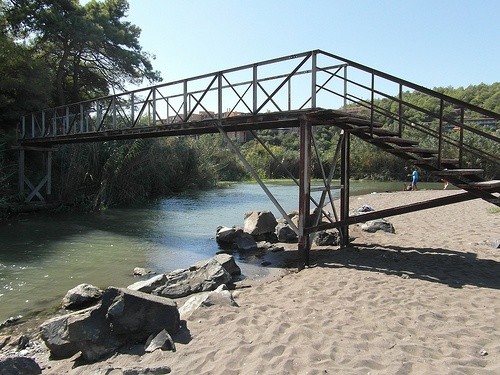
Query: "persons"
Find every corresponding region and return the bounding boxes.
[408,168,419,191]
[441,168,448,190]
